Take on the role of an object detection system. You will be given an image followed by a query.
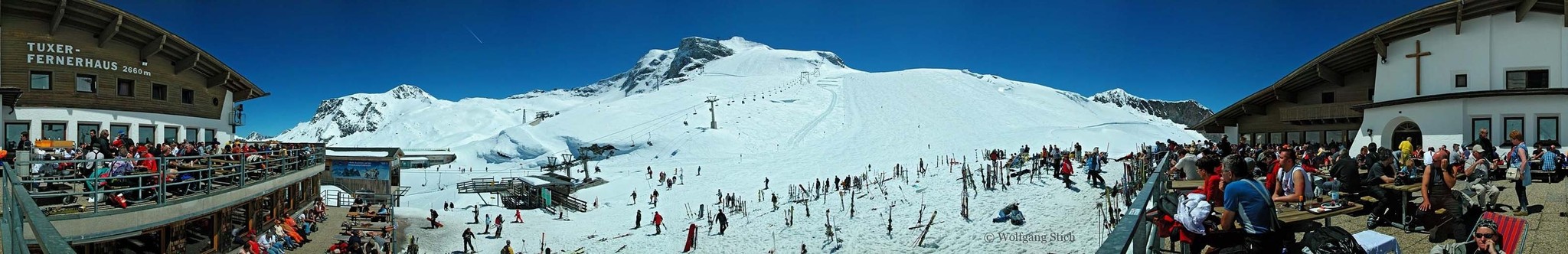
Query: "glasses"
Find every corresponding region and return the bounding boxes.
[1476,233,1495,238]
[1396,172,1405,176]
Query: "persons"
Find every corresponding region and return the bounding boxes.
[844,176,849,186]
[4,121,393,254]
[985,140,1033,188]
[429,159,783,254]
[868,163,871,171]
[992,201,1019,223]
[919,158,925,172]
[815,178,820,198]
[825,178,830,189]
[1032,126,1564,254]
[847,175,851,186]
[833,175,840,191]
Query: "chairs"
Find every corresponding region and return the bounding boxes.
[1466,211,1528,254]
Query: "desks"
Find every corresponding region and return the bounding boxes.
[125,154,306,203]
[1171,155,1562,231]
[47,152,63,160]
[333,204,391,243]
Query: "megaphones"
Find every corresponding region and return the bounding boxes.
[234,104,244,111]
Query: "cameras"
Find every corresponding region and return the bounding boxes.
[1479,172,1485,177]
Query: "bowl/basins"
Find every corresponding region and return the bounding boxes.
[61,170,70,175]
[40,173,46,177]
[29,175,39,177]
[177,154,183,157]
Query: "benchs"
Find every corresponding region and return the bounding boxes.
[66,168,79,172]
[105,182,130,189]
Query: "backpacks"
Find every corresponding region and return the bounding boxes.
[119,135,134,152]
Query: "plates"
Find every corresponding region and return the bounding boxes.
[105,162,109,164]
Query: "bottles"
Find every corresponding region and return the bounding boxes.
[272,150,274,155]
[175,151,177,157]
[290,150,292,156]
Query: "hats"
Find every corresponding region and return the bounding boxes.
[136,146,147,152]
[1472,144,1485,153]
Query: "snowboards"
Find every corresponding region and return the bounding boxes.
[427,217,443,227]
[758,189,761,202]
[684,223,695,252]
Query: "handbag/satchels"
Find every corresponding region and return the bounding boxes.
[1505,167,1520,181]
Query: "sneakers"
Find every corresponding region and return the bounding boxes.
[114,193,127,208]
[1513,207,1529,216]
[1366,213,1376,226]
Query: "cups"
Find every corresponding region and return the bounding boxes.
[191,160,193,167]
[78,206,86,212]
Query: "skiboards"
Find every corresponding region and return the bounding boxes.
[461,246,483,254]
[887,202,937,247]
[761,190,764,201]
[784,177,855,251]
[407,235,418,254]
[937,141,1074,222]
[685,196,747,235]
[534,250,557,254]
[510,221,524,223]
[570,233,634,254]
[853,164,929,195]
[1097,143,1153,251]
